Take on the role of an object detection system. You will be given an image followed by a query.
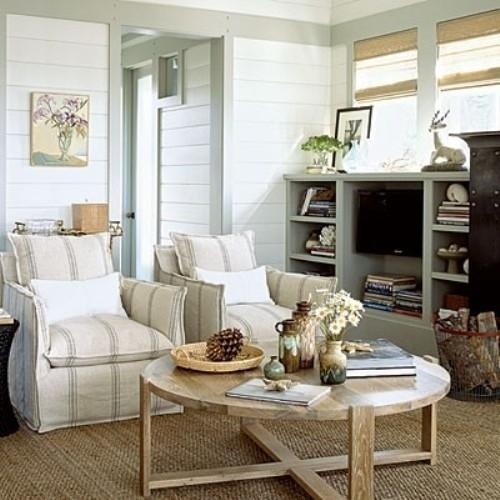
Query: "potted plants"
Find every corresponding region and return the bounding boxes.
[300,133,343,178]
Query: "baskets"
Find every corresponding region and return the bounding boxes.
[71,202,109,234]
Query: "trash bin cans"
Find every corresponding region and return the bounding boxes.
[433,316,500,402]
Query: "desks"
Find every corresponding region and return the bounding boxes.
[0,318,24,439]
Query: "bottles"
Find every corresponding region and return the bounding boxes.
[292,300,315,369]
[265,356,284,380]
[318,340,347,385]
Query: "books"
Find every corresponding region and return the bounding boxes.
[340,336,414,368]
[345,366,416,378]
[309,244,334,257]
[360,271,422,318]
[0,308,14,325]
[436,200,470,226]
[224,376,332,406]
[297,185,336,217]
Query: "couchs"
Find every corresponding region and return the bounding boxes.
[145,231,353,357]
[4,230,194,436]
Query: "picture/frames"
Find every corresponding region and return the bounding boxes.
[333,104,375,175]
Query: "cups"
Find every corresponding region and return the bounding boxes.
[274,319,301,373]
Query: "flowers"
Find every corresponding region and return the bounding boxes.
[298,284,366,344]
[32,92,89,150]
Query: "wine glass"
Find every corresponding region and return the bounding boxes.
[437,251,467,273]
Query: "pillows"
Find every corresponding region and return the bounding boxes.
[6,229,116,291]
[169,229,260,280]
[190,260,277,311]
[27,270,131,328]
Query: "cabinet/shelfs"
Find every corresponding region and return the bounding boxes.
[423,171,474,334]
[282,180,382,304]
[342,175,431,357]
[450,128,500,333]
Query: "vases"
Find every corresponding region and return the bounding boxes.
[317,338,350,386]
[264,355,286,380]
[56,126,73,161]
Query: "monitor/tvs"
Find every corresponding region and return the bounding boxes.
[357,189,423,257]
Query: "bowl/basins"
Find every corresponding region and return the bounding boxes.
[170,340,265,373]
[173,366,262,389]
